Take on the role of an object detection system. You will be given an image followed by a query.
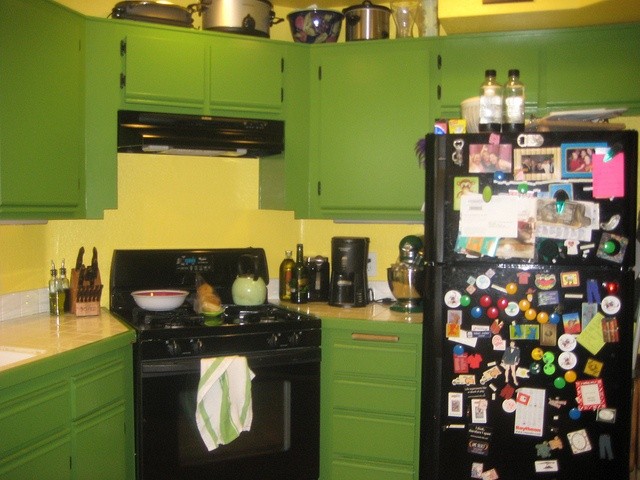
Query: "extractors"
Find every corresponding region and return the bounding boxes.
[117,110,285,158]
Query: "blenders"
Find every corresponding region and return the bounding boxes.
[386,235,424,313]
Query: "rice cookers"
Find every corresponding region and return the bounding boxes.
[188,0,283,37]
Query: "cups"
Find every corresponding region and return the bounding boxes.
[391,0,419,40]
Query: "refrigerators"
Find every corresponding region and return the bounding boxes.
[418,131,638,480]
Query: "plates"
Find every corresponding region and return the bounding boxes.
[204,317,224,327]
[198,309,225,317]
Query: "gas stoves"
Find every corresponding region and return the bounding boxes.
[111,305,320,330]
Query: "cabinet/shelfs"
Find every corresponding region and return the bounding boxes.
[438,24,640,121]
[0,1,88,222]
[319,315,422,480]
[0,311,138,480]
[122,23,286,123]
[310,37,434,215]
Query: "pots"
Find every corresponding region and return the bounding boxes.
[343,1,392,40]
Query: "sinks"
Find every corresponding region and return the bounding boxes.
[0,341,47,373]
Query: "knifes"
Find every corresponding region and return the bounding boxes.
[76,247,103,302]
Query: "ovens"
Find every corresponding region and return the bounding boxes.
[132,347,322,480]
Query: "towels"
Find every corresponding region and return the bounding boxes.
[194,355,256,454]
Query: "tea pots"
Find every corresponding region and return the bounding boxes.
[231,254,267,307]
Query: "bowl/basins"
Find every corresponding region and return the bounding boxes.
[287,4,344,44]
[130,289,189,312]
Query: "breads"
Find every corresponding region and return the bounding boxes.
[197,284,220,312]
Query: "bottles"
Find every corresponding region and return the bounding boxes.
[291,243,308,303]
[504,69,526,132]
[59,260,69,314]
[48,261,59,315]
[279,251,295,300]
[478,70,501,131]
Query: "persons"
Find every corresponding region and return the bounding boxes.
[522,157,554,173]
[469,148,512,171]
[567,149,596,171]
[501,341,521,386]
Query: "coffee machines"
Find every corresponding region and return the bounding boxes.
[329,237,369,308]
